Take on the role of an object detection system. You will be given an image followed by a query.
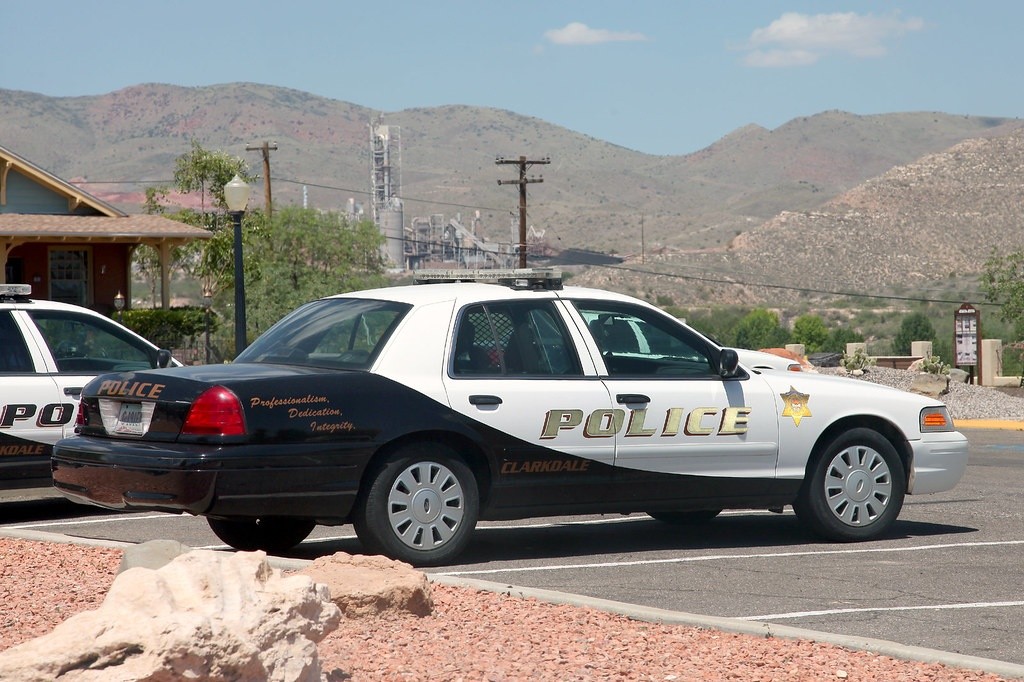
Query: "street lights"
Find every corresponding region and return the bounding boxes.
[223,172,252,360]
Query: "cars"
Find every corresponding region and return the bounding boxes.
[0,283,187,472]
[51,283,968,566]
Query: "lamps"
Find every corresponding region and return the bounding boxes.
[114,290,127,310]
[33,271,42,284]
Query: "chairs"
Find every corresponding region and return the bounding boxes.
[504,322,542,372]
